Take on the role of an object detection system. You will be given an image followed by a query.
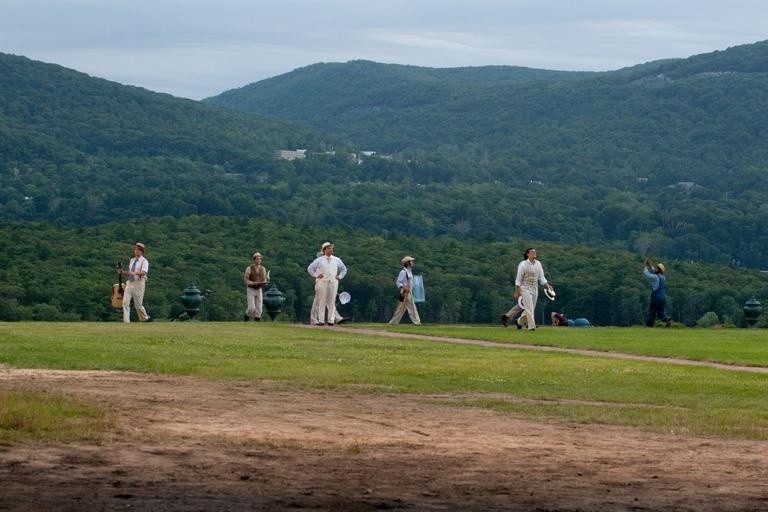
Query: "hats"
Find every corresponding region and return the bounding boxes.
[544,288,555,301]
[134,243,145,252]
[656,263,666,273]
[518,296,525,310]
[253,252,263,259]
[401,256,415,263]
[321,242,334,252]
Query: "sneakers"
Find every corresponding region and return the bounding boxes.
[316,318,349,326]
[515,319,522,329]
[665,318,672,327]
[501,315,509,327]
[242,313,261,321]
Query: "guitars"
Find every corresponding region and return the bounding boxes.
[110,261,126,308]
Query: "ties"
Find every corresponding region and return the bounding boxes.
[129,259,138,282]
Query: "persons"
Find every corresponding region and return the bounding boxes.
[642,258,673,329]
[118,242,151,323]
[501,252,540,329]
[551,312,569,326]
[307,241,348,326]
[388,256,422,326]
[309,251,351,325]
[243,252,268,322]
[513,247,553,331]
